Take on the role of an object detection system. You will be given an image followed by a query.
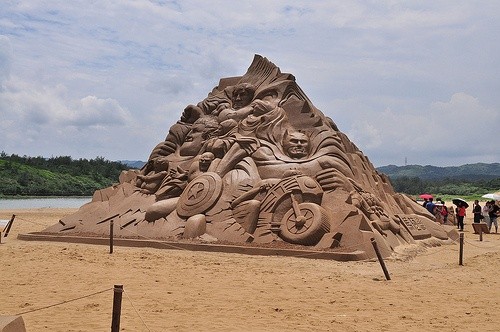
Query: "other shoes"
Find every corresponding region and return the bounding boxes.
[459,229,463,231]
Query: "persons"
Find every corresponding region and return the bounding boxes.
[457,203,465,231]
[423,198,429,207]
[473,200,481,233]
[488,200,500,233]
[483,201,491,228]
[434,197,448,224]
[426,198,435,213]
[137,83,355,223]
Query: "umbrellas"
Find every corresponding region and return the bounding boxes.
[452,198,469,209]
[418,194,432,199]
[482,194,500,200]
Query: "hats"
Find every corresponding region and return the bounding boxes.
[434,201,443,206]
